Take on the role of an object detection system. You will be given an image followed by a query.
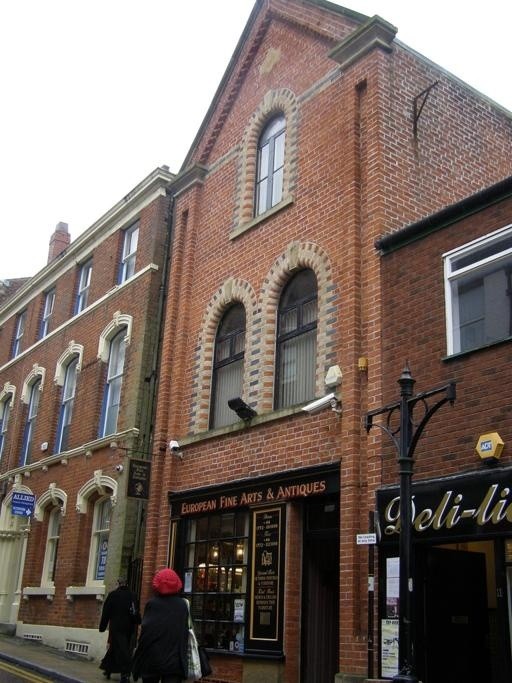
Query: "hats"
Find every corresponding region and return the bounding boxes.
[152,567,182,595]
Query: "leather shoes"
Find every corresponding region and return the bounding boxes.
[120,677,129,683]
[103,671,110,680]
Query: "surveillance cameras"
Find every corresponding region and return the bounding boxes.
[115,464,123,472]
[300,392,337,414]
[168,441,180,457]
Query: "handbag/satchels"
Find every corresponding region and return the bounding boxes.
[130,606,141,625]
[188,626,212,683]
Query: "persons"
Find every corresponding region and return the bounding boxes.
[98,576,142,682]
[131,568,194,681]
